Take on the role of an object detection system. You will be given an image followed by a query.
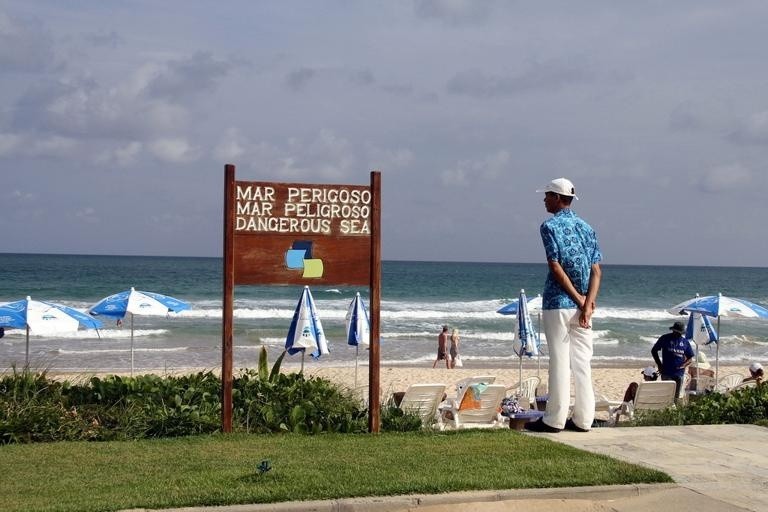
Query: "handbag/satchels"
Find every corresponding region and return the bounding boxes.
[454,357,462,367]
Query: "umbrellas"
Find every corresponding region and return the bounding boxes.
[344,291,384,389]
[89,286,193,377]
[512,288,540,395]
[684,292,719,370]
[283,285,330,381]
[0,296,103,376]
[665,291,768,393]
[496,294,546,375]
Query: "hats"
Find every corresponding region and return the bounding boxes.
[669,322,686,334]
[689,351,711,369]
[443,325,449,330]
[749,363,764,372]
[641,367,659,378]
[536,177,579,200]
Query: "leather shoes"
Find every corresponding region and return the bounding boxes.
[524,416,589,433]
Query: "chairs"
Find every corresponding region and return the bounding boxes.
[390,375,542,429]
[593,376,765,427]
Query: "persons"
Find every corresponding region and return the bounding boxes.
[523,178,604,433]
[116,318,122,327]
[432,325,451,368]
[449,329,461,369]
[651,321,695,402]
[683,352,715,391]
[610,367,658,416]
[741,362,766,388]
